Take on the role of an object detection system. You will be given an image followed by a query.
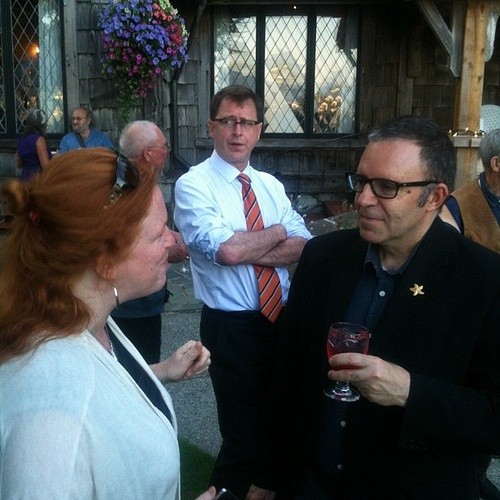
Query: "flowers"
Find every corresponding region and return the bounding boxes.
[93,1,190,129]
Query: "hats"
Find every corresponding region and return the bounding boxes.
[237,173,282,324]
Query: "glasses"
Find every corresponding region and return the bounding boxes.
[344,171,438,199]
[103,150,139,209]
[148,144,172,153]
[72,117,82,121]
[214,118,261,131]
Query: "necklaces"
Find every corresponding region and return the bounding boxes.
[87,324,117,360]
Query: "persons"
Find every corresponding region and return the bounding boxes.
[175,86,311,500]
[1,107,187,365]
[0,149,216,500]
[248,122,500,500]
[441,129,499,252]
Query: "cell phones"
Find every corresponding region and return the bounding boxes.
[215,488,238,500]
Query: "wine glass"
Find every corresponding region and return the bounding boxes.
[323,322,369,402]
